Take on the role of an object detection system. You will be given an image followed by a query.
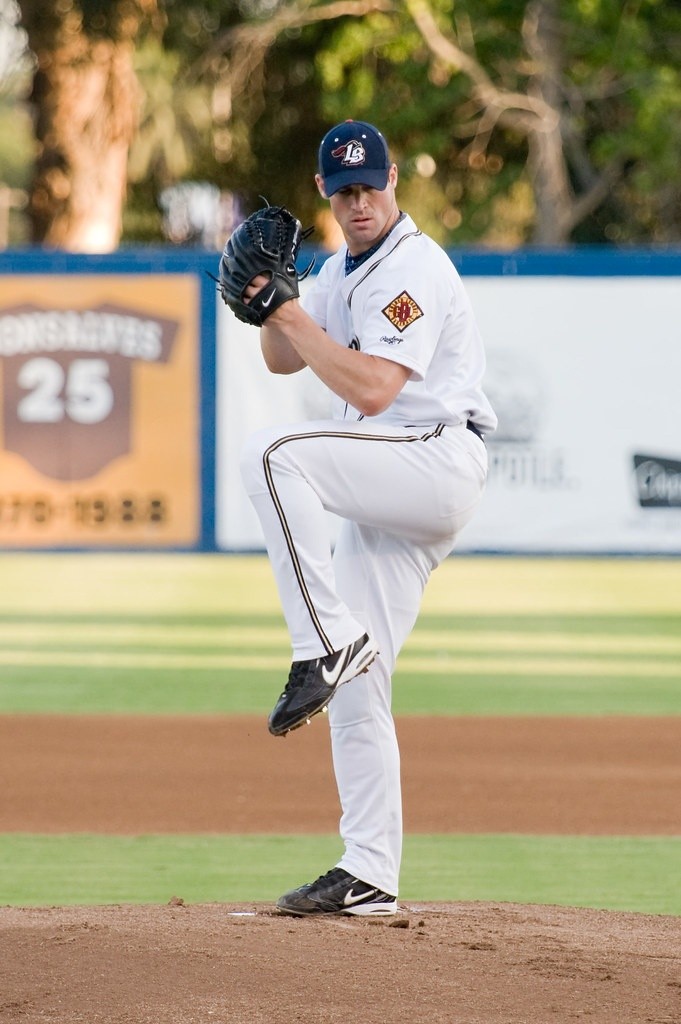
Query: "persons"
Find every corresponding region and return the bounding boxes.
[218,120,497,916]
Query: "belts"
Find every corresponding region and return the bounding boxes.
[466,418,485,444]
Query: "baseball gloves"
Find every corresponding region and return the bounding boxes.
[222,204,300,326]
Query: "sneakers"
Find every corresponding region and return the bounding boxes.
[267,628,379,737]
[277,868,398,917]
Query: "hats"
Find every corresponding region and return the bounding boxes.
[320,119,391,197]
